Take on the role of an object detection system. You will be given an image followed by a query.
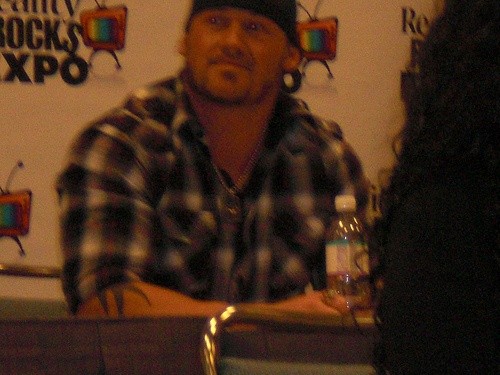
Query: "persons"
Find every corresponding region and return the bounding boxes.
[370,0,500,374]
[57,0,374,331]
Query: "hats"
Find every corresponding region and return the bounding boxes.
[185,0,298,47]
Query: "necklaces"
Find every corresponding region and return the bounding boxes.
[183,74,279,216]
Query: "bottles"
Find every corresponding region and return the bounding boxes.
[326,195,375,328]
